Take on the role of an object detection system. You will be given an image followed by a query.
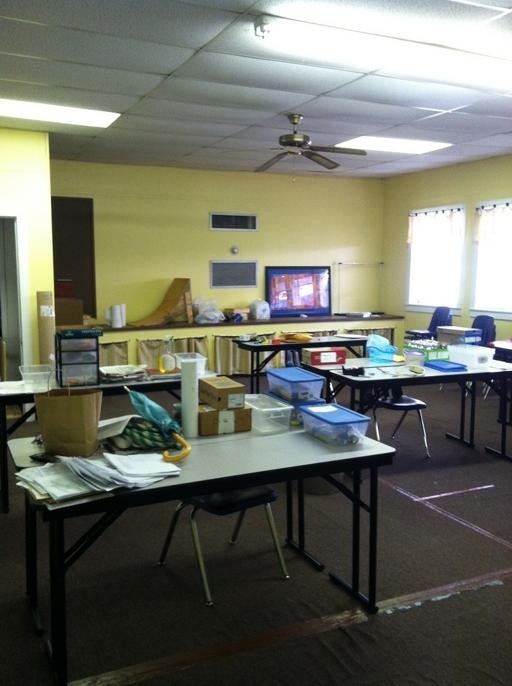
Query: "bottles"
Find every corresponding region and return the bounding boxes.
[158,335,176,373]
[111,304,127,328]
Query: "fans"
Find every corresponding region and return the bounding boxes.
[255,114,367,173]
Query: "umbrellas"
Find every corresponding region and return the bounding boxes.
[123,385,192,462]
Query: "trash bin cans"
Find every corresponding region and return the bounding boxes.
[19,365,55,422]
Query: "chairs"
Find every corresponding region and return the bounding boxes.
[471,315,497,349]
[158,486,290,606]
[370,383,431,463]
[403,306,451,343]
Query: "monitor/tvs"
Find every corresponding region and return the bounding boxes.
[266,266,331,318]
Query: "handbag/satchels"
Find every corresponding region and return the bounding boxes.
[34,387,103,457]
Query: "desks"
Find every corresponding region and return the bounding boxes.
[1,368,217,515]
[6,432,397,685]
[303,356,512,462]
[234,334,367,400]
